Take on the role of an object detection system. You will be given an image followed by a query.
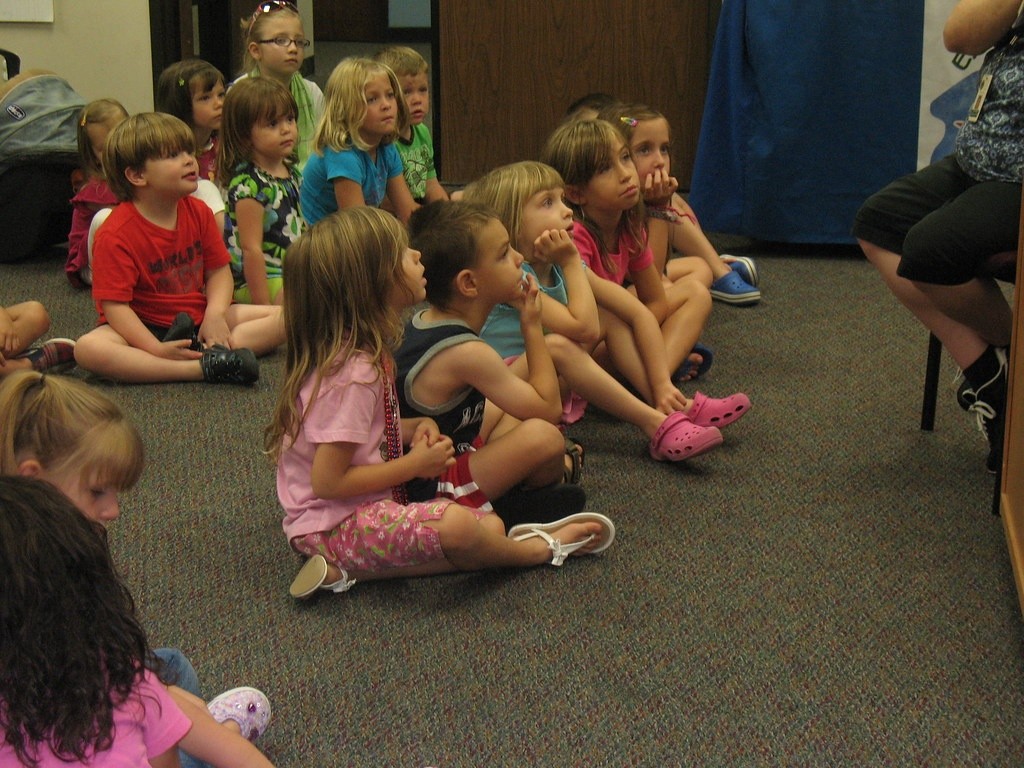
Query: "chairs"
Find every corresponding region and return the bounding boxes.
[921,259,1020,516]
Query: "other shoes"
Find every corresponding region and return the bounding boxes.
[206,687,272,742]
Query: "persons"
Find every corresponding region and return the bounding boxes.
[63,88,64,92]
[298,58,424,232]
[0,301,76,377]
[153,58,226,183]
[392,199,587,537]
[566,93,761,306]
[0,474,194,768]
[596,91,712,299]
[461,160,752,462]
[226,0,326,173]
[73,111,288,384]
[370,46,450,229]
[65,98,226,289]
[261,205,616,598]
[852,0,1024,474]
[538,119,713,381]
[223,76,310,306]
[0,369,272,767]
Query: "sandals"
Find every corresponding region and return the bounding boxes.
[563,436,586,483]
[720,253,759,287]
[492,479,587,528]
[508,512,616,566]
[709,270,761,306]
[671,346,713,383]
[290,553,355,599]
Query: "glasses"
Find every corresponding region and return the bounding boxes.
[248,0,300,34]
[257,36,311,49]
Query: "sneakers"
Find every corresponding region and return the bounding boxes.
[162,311,197,351]
[200,342,260,387]
[957,345,1009,472]
[15,337,76,373]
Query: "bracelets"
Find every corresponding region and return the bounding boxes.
[646,205,696,225]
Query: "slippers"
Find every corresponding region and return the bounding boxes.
[687,390,750,429]
[649,411,724,461]
